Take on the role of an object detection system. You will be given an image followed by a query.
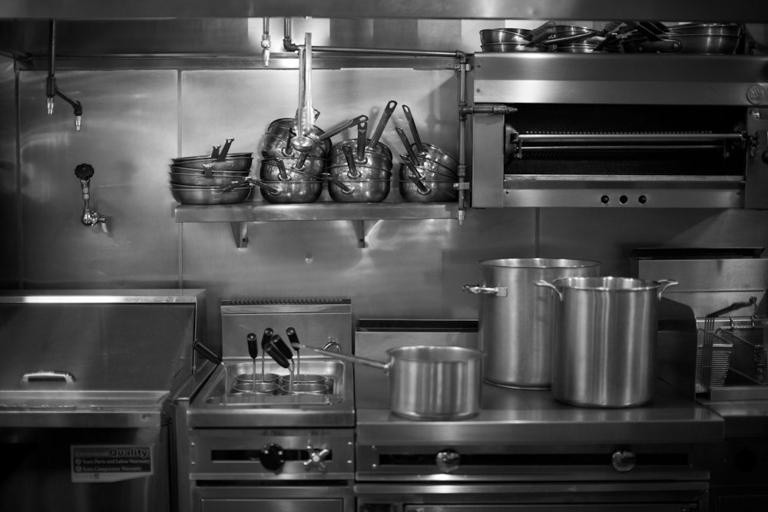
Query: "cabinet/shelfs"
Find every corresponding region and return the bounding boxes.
[171,55,768,253]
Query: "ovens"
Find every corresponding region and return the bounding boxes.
[469,78,767,211]
[354,420,726,512]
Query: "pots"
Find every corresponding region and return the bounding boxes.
[290,342,488,421]
[459,255,602,391]
[167,136,254,205]
[479,15,744,56]
[531,274,681,408]
[261,103,458,203]
